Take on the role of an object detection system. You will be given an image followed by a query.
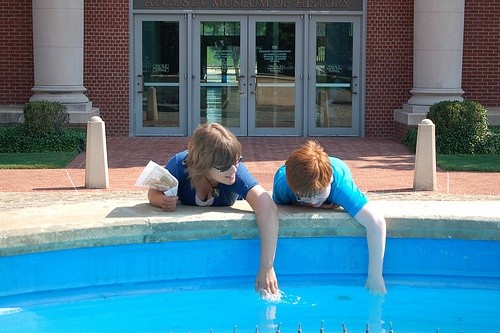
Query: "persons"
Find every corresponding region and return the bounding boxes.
[270,139,387,295]
[145,120,280,299]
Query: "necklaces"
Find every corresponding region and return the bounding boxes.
[203,172,221,198]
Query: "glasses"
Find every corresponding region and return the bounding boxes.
[213,155,242,172]
[295,188,328,202]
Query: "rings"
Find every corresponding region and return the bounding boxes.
[167,205,170,209]
